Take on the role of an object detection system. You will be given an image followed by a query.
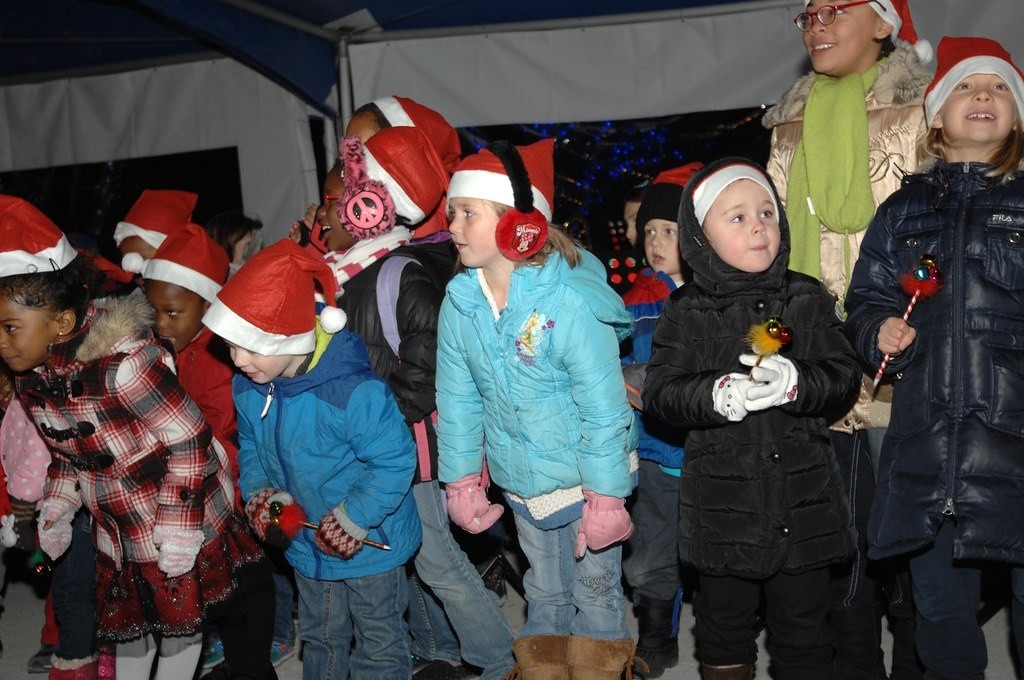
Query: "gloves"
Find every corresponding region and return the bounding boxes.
[713,341,799,421]
[621,363,647,412]
[445,473,504,534]
[574,487,635,559]
[314,505,370,562]
[244,487,304,549]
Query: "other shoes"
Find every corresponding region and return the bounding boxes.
[202,636,225,668]
[621,643,679,680]
[412,659,467,680]
[270,641,296,668]
[509,634,650,680]
[27,643,61,672]
[484,564,508,608]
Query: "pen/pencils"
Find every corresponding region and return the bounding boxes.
[269,501,391,551]
[870,256,937,398]
[748,317,788,382]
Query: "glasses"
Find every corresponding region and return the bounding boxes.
[794,0,887,32]
[324,193,343,208]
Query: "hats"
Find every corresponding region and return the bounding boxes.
[141,223,230,303]
[74,247,144,294]
[113,189,198,249]
[923,34,1024,127]
[804,0,933,63]
[360,94,554,223]
[620,145,781,226]
[0,194,79,278]
[201,238,347,355]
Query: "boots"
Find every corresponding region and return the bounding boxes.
[621,587,684,680]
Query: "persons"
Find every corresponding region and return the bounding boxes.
[839,35,1024,680]
[1,93,861,680]
[764,1,939,680]
